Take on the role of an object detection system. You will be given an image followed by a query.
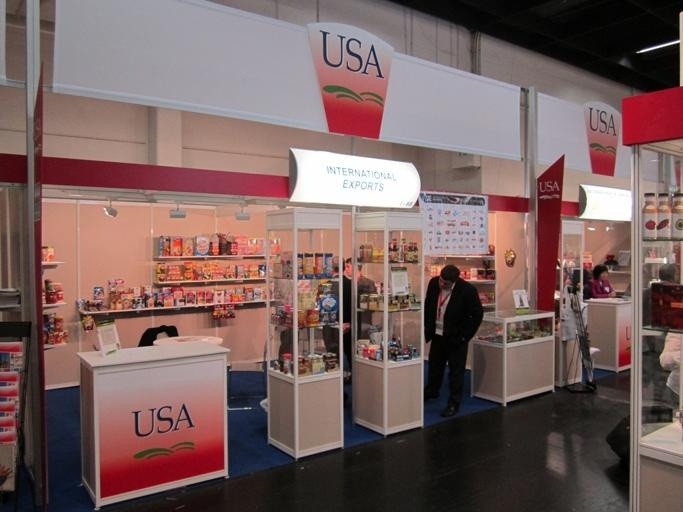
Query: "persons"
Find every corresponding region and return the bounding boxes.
[423,263,483,416]
[661,326,682,412]
[317,255,356,382]
[641,264,677,416]
[589,265,616,299]
[572,268,592,300]
[345,257,379,341]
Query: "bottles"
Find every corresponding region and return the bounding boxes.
[358,238,419,263]
[642,191,683,242]
[297,252,336,277]
[270,304,306,329]
[359,293,416,311]
[357,341,417,362]
[282,353,292,374]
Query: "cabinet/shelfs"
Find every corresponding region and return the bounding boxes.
[41,255,273,349]
[620,87,682,512]
[268,208,422,459]
[427,254,556,407]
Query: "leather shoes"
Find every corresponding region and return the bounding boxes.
[441,405,458,417]
[424,388,440,401]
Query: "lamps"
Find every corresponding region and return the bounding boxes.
[102,198,251,219]
